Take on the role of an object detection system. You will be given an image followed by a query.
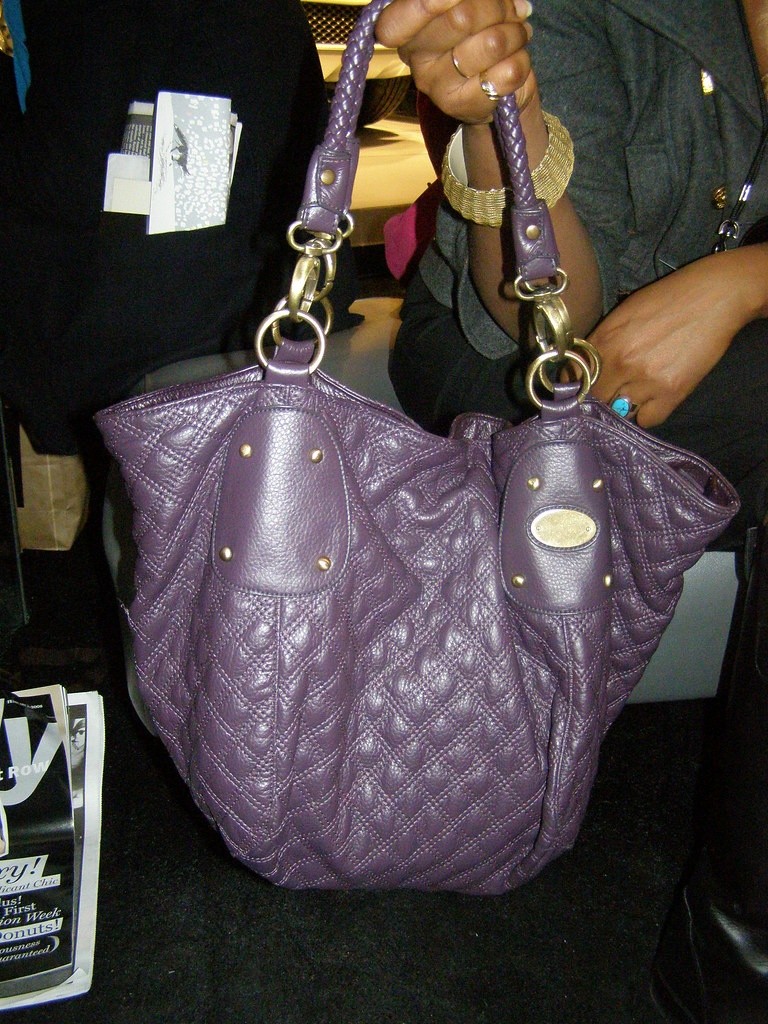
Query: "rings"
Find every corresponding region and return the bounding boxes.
[451,47,471,79]
[479,70,502,102]
[611,395,640,419]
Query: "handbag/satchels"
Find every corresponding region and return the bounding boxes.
[651,886,768,1024]
[90,0,741,898]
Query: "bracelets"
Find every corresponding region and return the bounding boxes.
[439,110,575,229]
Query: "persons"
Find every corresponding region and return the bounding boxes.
[385,0,768,551]
[0,0,330,665]
